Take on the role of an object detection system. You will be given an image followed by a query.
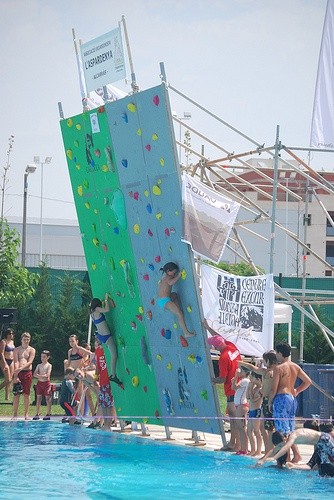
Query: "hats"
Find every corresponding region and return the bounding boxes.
[207,335,225,348]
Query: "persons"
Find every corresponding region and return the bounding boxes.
[285,422,334,477]
[158,262,196,337]
[230,363,250,455]
[57,334,136,430]
[203,318,242,452]
[255,428,334,469]
[33,350,53,420]
[91,291,122,384]
[0,328,15,400]
[246,371,264,456]
[268,342,312,464]
[237,351,277,459]
[10,331,35,421]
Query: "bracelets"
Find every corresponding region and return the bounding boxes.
[104,300,109,301]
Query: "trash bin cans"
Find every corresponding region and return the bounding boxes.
[292,363,334,418]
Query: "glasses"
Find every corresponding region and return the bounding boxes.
[7,333,13,335]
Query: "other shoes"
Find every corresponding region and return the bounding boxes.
[108,375,123,384]
[88,423,98,428]
[222,443,235,450]
[43,414,51,420]
[111,421,116,427]
[33,414,40,420]
[74,421,81,425]
